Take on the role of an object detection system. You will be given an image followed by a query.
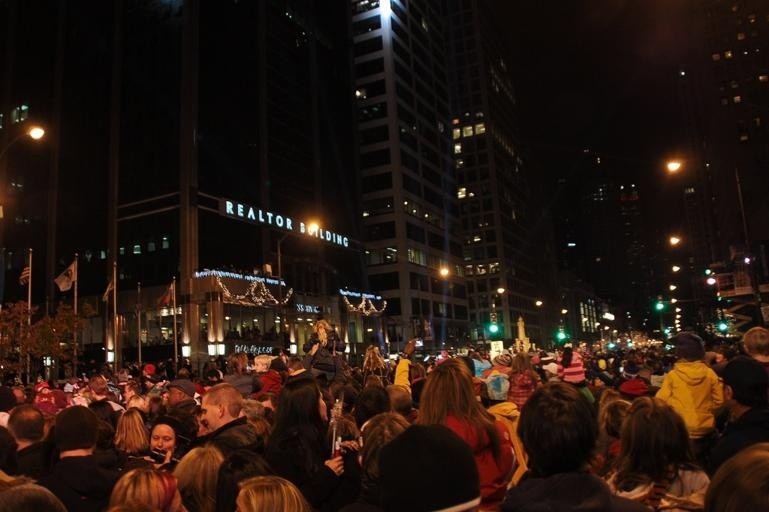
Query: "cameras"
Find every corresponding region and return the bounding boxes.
[151,450,183,464]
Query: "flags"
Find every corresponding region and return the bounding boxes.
[19,254,30,285]
[54,260,76,292]
[157,282,174,307]
[103,268,114,304]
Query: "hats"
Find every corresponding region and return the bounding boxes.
[55,403,101,452]
[618,380,650,396]
[378,424,482,511]
[165,379,196,397]
[144,364,156,376]
[485,353,514,402]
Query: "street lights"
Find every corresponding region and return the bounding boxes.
[666,154,764,326]
[416,265,449,322]
[0,124,45,164]
[276,221,319,353]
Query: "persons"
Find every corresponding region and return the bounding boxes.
[0,319,769,512]
